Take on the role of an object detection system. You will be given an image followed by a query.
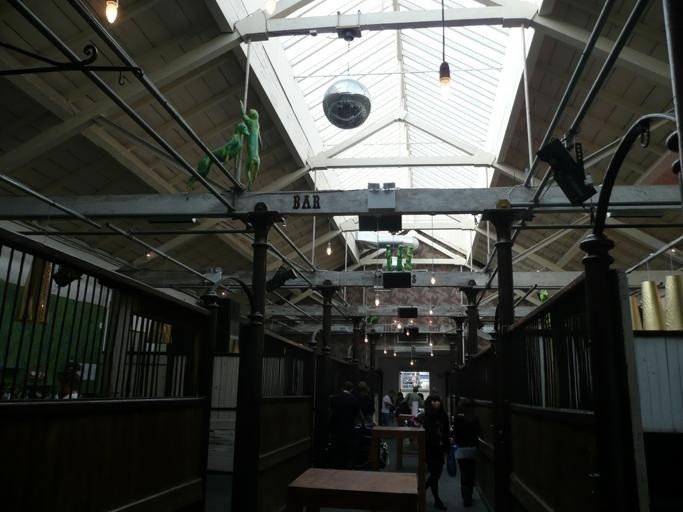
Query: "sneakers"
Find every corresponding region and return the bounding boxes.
[434,503,447,510]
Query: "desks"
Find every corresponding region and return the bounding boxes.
[289,467,431,512]
[397,413,422,469]
[352,425,425,512]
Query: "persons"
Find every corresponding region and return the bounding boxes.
[56,371,82,399]
[326,379,483,510]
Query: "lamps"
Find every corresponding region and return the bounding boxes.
[438,0,450,84]
[322,28,371,128]
[105,0,119,23]
[325,208,437,365]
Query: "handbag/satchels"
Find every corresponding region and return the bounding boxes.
[447,453,456,477]
